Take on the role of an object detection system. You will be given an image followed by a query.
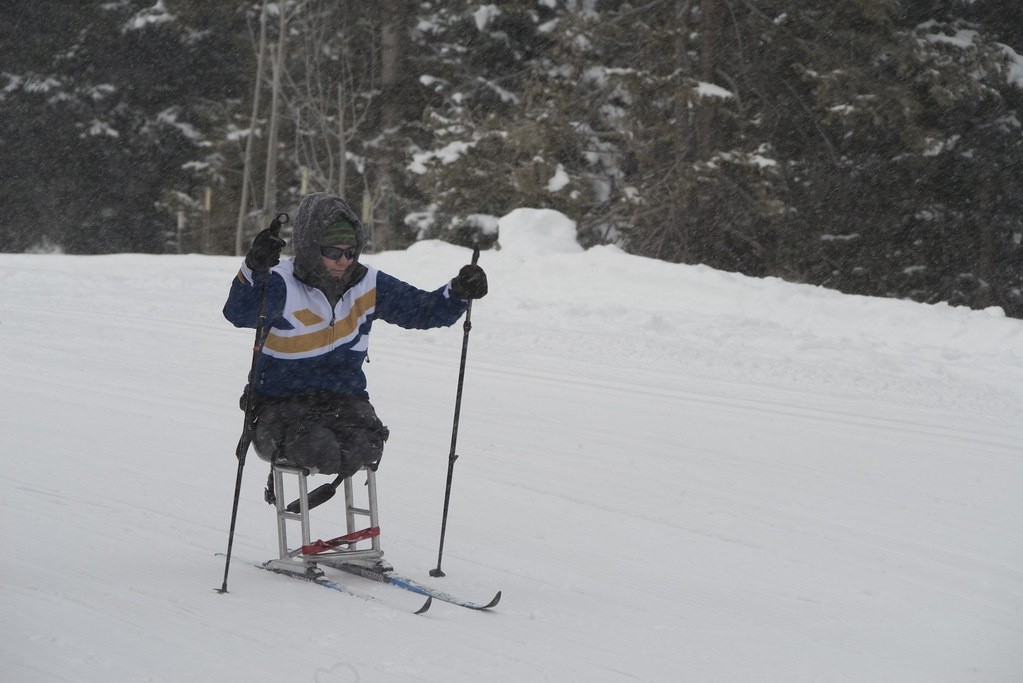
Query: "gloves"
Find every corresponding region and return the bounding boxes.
[245,228,286,273]
[451,264,488,300]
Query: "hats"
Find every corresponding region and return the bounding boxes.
[320,217,358,246]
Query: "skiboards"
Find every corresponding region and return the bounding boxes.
[212,528,502,615]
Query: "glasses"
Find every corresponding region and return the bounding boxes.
[320,246,357,260]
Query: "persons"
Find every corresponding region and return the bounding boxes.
[222,192,488,474]
